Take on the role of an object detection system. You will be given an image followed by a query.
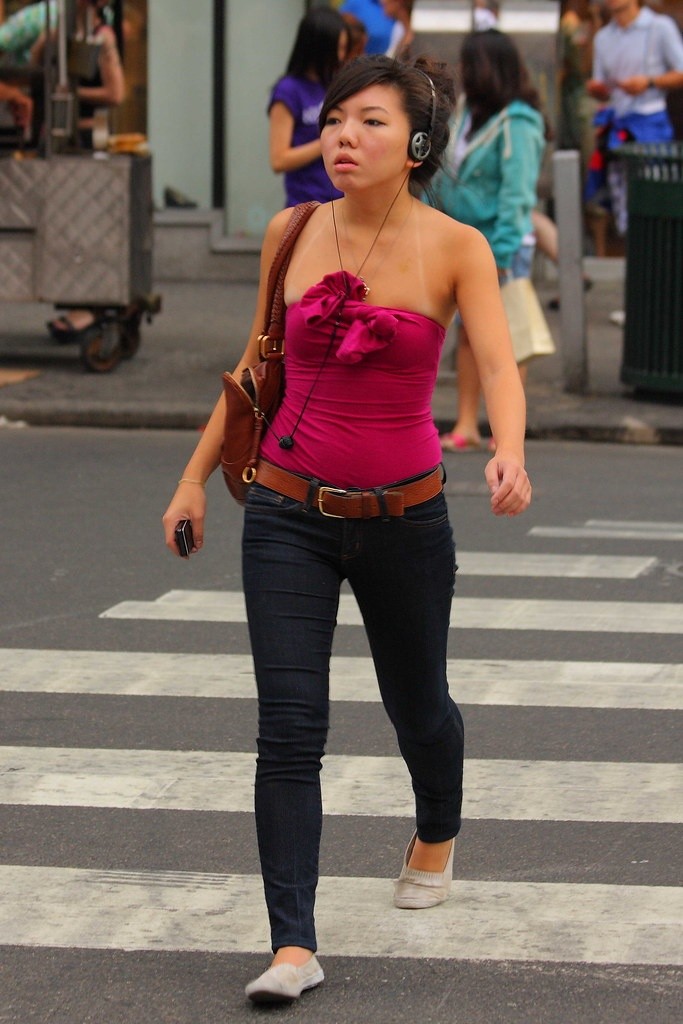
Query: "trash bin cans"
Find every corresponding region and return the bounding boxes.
[606,141,683,400]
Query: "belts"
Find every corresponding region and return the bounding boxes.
[254,457,443,519]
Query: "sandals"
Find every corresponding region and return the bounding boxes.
[46,308,105,341]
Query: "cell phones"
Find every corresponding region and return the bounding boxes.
[175,519,194,557]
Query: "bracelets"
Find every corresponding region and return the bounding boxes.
[649,77,654,88]
[177,478,206,487]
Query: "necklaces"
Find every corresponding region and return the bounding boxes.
[340,196,414,302]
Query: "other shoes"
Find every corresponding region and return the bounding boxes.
[393,829,455,908]
[244,952,326,998]
[550,276,594,310]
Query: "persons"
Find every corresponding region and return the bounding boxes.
[265,0,558,454]
[585,0,683,325]
[160,54,534,1005]
[29,1,127,344]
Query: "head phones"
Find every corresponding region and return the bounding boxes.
[407,68,437,163]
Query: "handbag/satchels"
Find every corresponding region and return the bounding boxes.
[500,269,555,365]
[220,201,320,506]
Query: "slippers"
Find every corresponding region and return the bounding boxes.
[488,437,497,453]
[438,433,482,453]
